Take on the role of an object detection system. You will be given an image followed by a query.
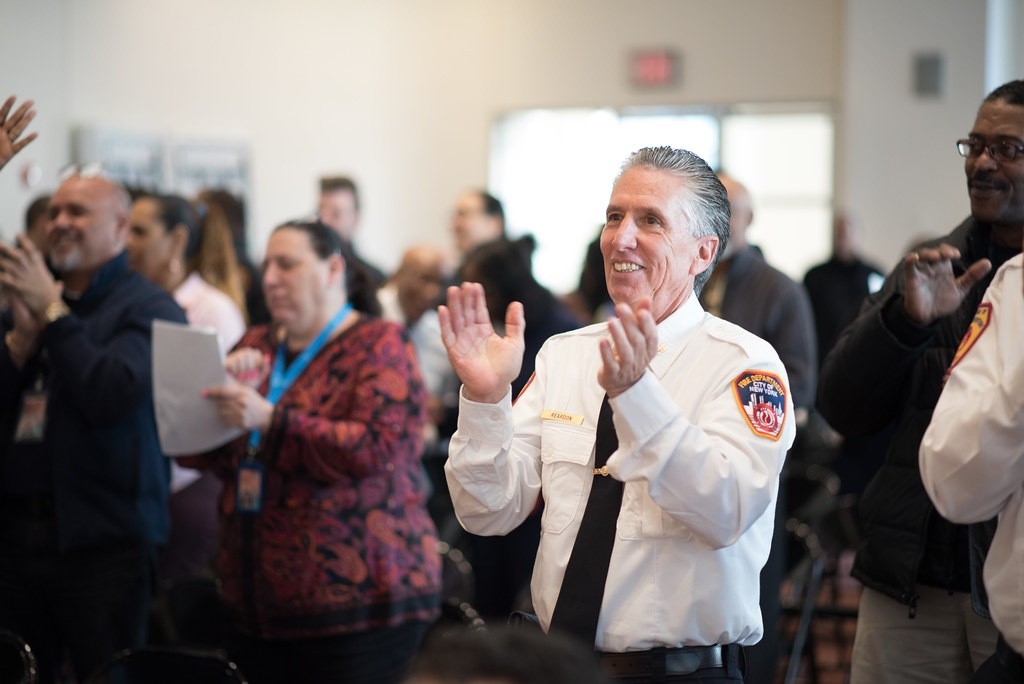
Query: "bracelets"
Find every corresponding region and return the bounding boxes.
[41,303,70,324]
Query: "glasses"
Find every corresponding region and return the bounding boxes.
[956,138,1024,162]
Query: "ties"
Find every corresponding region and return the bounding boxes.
[546,390,625,648]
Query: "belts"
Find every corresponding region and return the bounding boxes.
[591,643,725,678]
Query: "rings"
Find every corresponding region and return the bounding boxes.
[8,131,16,141]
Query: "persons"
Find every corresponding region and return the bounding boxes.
[0,77,1024,684]
